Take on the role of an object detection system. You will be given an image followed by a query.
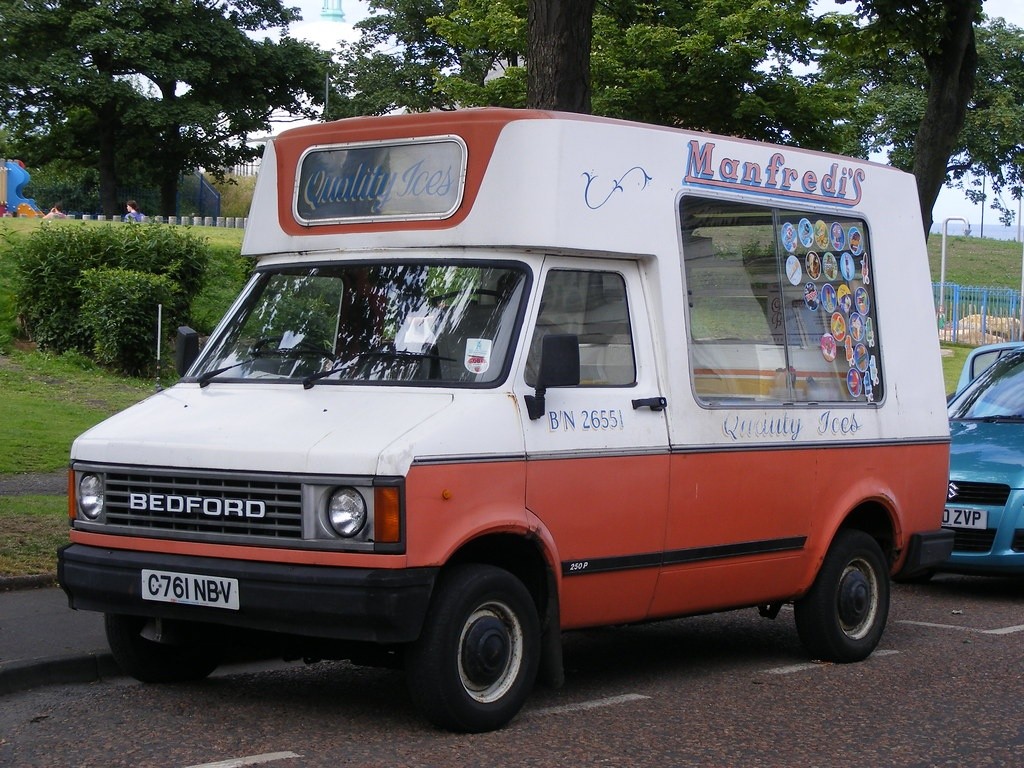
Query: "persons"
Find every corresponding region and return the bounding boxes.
[937,305,947,335]
[42,204,67,219]
[491,276,570,387]
[123,200,146,222]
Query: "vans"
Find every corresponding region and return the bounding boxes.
[53,105,956,740]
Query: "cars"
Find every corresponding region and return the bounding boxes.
[942,341,1024,578]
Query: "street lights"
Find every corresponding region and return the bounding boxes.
[939,217,973,327]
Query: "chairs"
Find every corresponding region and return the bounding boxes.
[429,289,502,381]
